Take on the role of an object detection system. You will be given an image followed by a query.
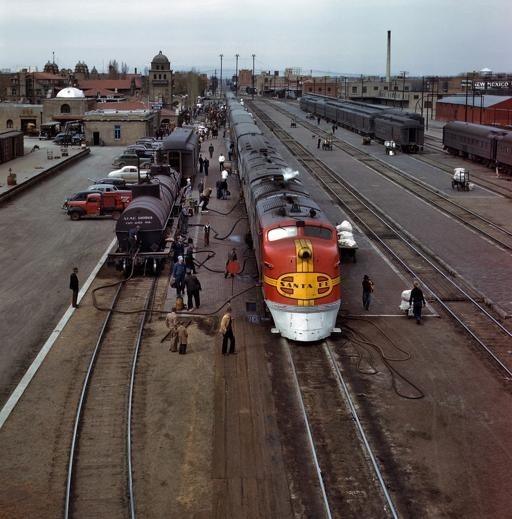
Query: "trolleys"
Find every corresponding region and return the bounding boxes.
[400,305,425,319]
[321,138,332,150]
[384,142,396,155]
[451,170,470,191]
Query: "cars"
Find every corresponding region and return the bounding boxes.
[55,133,84,143]
[62,137,164,210]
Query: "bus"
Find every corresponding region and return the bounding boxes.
[39,121,61,140]
[65,121,82,133]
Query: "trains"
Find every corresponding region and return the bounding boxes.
[299,92,425,153]
[108,127,200,276]
[442,120,512,175]
[225,89,342,342]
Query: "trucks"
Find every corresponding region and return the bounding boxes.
[66,190,132,220]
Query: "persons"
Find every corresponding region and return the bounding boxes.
[164,306,179,353]
[203,221,211,247]
[128,224,141,260]
[69,267,80,308]
[228,145,234,161]
[200,190,211,214]
[203,157,209,176]
[155,99,227,141]
[174,321,188,356]
[173,254,187,298]
[361,273,374,312]
[182,270,203,310]
[220,306,237,356]
[222,178,228,200]
[198,154,204,173]
[174,235,200,274]
[198,178,204,205]
[209,143,214,159]
[215,178,223,200]
[407,281,425,325]
[219,153,226,171]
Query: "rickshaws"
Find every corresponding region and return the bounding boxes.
[335,225,358,257]
